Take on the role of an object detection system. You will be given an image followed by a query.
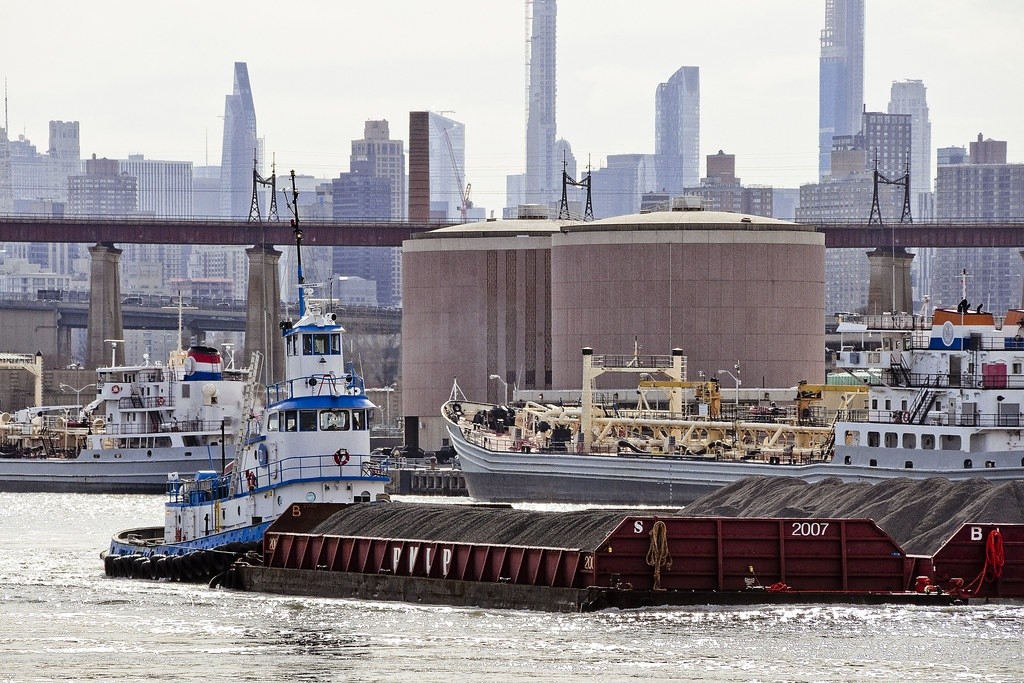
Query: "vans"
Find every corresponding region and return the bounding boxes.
[121,297,143,306]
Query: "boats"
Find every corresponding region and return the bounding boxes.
[109,170,392,556]
[0,290,265,494]
[440,269,1024,512]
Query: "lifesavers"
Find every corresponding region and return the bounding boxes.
[247,472,256,488]
[158,396,164,405]
[454,404,462,411]
[334,450,350,465]
[258,442,267,467]
[111,385,120,393]
[105,542,241,581]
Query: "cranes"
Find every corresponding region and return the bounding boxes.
[443,127,473,217]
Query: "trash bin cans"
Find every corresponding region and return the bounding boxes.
[435,451,449,464]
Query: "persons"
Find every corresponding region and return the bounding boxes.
[171,417,177,430]
[612,392,622,419]
[472,411,483,431]
